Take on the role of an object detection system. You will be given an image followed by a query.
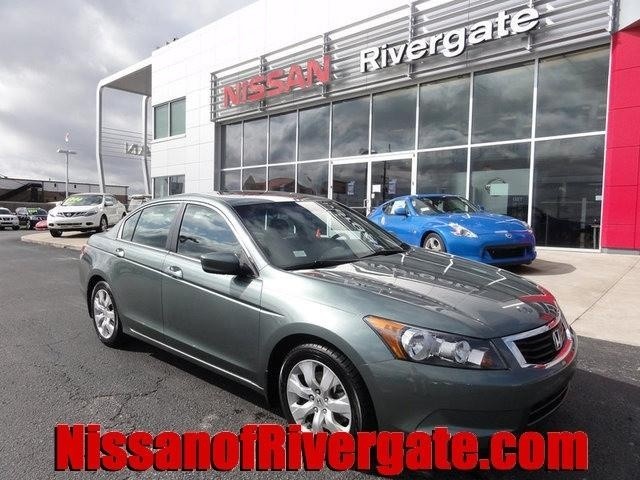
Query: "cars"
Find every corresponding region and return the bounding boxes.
[45,191,128,239]
[76,190,580,475]
[33,219,47,231]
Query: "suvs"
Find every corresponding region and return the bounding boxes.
[0,206,20,231]
[15,207,50,230]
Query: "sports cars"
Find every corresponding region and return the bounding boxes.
[364,192,539,270]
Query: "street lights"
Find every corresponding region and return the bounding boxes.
[57,148,79,199]
[361,148,378,189]
